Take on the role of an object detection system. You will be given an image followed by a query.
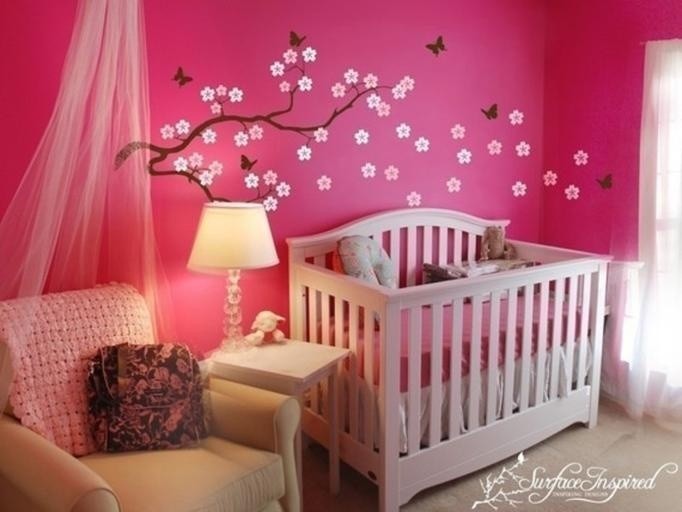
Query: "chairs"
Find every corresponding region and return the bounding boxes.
[1,281,298,511]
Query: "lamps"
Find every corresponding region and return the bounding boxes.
[187,201,282,355]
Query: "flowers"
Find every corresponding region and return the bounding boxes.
[210,334,349,512]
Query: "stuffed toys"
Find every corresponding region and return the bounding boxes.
[479,225,515,261]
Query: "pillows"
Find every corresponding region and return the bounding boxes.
[333,235,399,331]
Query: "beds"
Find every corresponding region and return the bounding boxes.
[281,205,616,511]
